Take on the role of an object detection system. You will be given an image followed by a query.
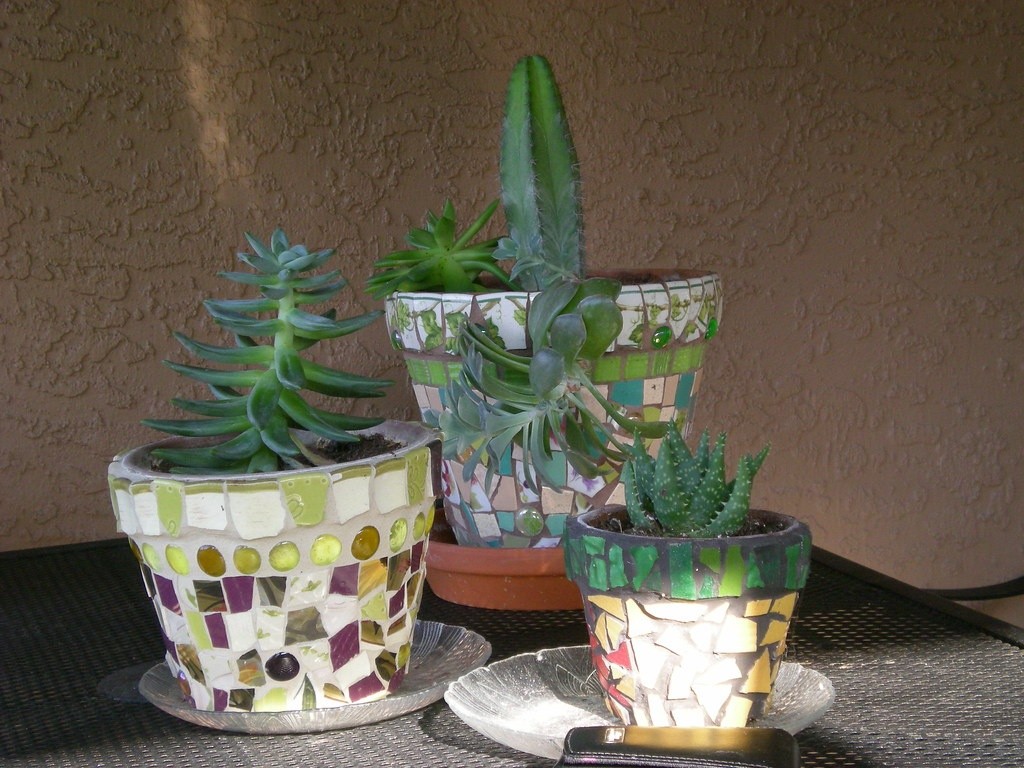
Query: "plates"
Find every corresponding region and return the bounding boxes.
[443,645,836,759]
[423,508,584,610]
[136,620,492,738]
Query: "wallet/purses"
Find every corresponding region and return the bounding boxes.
[553,726,800,768]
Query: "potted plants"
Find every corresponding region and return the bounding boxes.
[361,54,723,549]
[108,227,444,712]
[566,420,812,732]
